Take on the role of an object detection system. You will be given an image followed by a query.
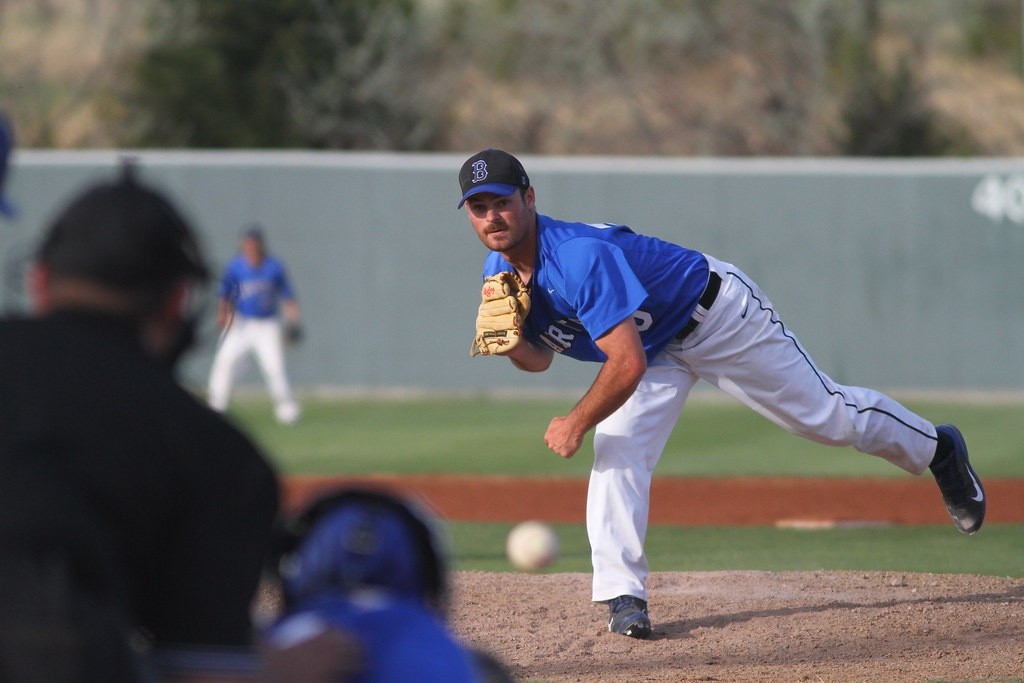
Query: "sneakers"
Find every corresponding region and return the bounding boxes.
[608,594,651,637]
[929,424,987,534]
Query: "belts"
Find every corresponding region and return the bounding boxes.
[674,271,722,340]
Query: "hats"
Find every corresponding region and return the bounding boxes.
[457,148,530,210]
[39,168,212,284]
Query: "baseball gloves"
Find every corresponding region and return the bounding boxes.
[470,272,531,354]
[282,320,305,347]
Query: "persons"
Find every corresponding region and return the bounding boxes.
[1,174,511,683]
[455,148,986,637]
[208,229,299,422]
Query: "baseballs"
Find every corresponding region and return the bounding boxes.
[504,521,561,572]
